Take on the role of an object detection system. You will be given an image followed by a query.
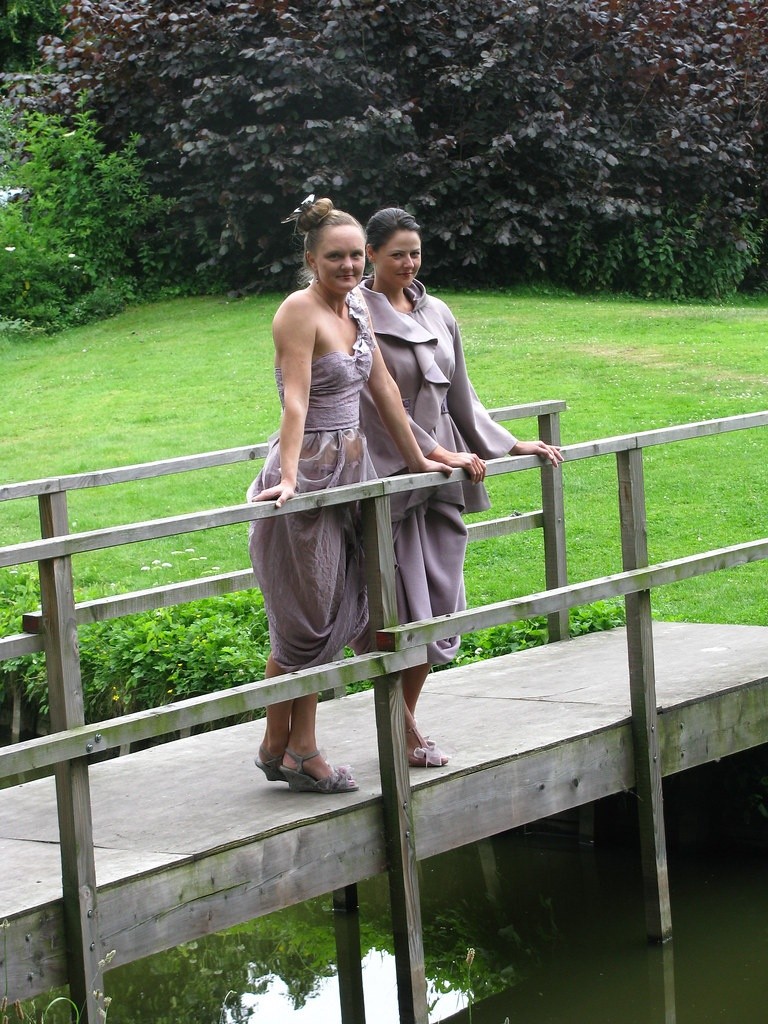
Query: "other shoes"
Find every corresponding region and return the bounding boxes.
[406,721,449,767]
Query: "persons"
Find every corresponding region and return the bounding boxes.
[246,195,453,793]
[358,208,564,768]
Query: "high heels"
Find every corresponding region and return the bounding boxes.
[278,748,359,794]
[255,746,288,782]
